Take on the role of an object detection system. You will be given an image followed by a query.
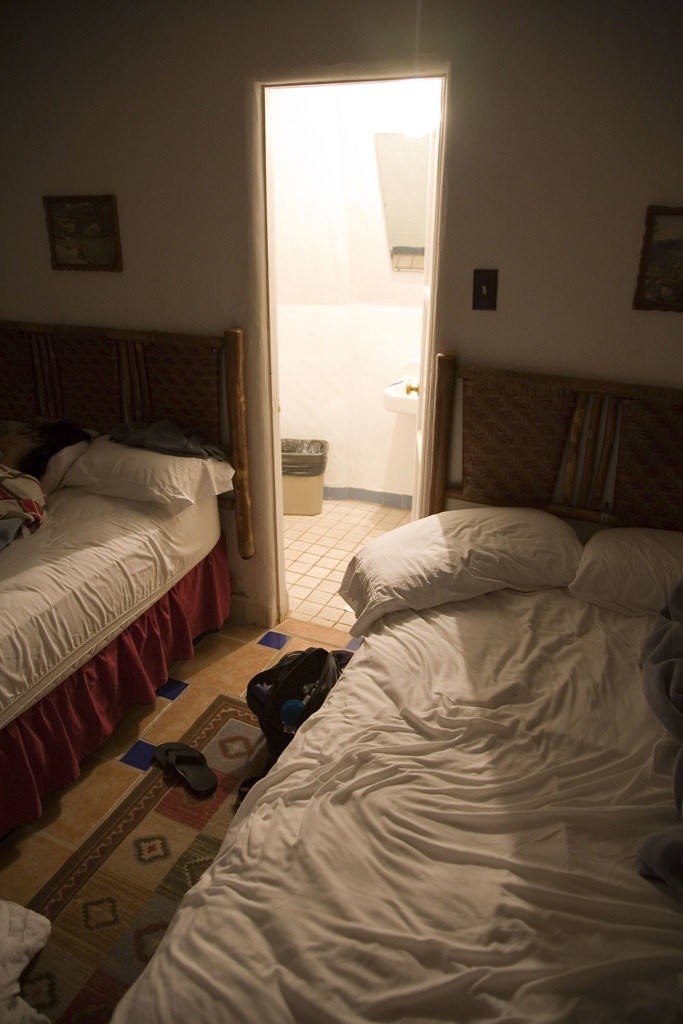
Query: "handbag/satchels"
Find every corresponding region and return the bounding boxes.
[245,647,353,763]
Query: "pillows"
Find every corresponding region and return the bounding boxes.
[567,526,683,617]
[337,503,584,640]
[56,433,236,515]
[1,420,101,495]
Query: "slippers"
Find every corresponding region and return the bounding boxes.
[238,776,264,800]
[154,742,218,796]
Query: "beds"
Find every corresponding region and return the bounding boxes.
[135,353,682,1023]
[1,319,254,838]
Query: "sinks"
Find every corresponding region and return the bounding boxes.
[384,376,419,416]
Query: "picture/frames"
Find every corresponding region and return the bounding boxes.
[632,205,683,313]
[42,193,124,273]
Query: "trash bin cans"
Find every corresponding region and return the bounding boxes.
[280,438,329,515]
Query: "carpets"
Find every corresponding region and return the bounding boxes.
[18,693,274,1024]
